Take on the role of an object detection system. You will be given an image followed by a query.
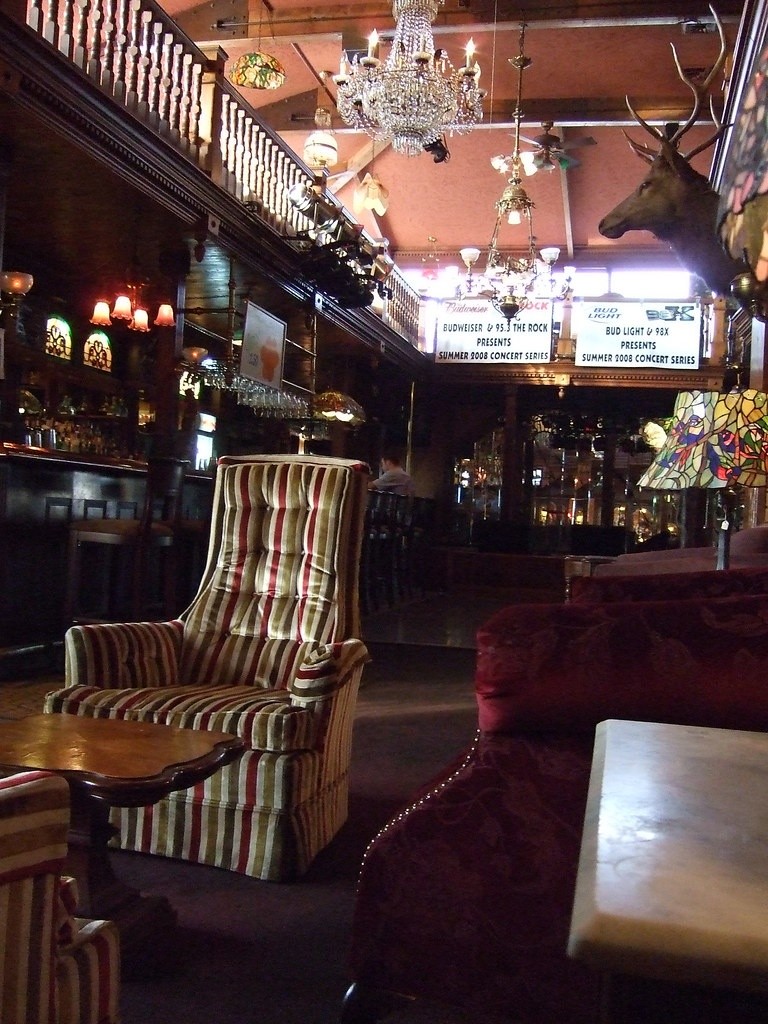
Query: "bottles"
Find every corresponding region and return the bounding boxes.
[57,395,127,417]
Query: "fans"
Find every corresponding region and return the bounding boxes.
[509,121,597,168]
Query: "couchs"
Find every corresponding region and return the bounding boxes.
[337,520,768,1020]
[42,451,374,884]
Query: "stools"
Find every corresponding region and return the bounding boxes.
[54,453,192,664]
[357,485,438,616]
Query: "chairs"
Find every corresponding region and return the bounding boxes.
[1,767,122,1024]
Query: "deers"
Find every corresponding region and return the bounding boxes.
[596,5,768,325]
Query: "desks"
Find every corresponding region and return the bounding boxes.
[561,716,768,1001]
[0,711,246,980]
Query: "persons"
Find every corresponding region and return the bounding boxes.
[368,447,414,495]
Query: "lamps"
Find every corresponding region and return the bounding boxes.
[455,21,575,329]
[1,271,33,337]
[331,0,488,159]
[280,181,394,311]
[635,386,768,569]
[315,383,377,428]
[229,0,287,90]
[354,135,391,216]
[88,274,176,332]
[181,346,210,383]
[304,71,336,168]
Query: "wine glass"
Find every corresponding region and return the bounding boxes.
[205,367,311,421]
